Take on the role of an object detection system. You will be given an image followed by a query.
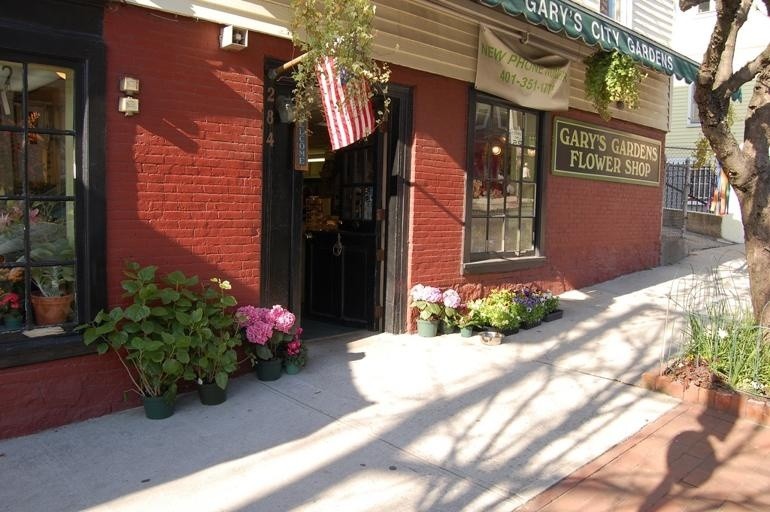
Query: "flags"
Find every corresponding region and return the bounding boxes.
[315,38,375,151]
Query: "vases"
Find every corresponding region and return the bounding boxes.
[255,357,303,381]
[542,309,564,322]
[484,325,520,336]
[415,318,473,338]
[3,312,22,328]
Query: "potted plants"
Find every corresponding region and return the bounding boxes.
[29,242,242,420]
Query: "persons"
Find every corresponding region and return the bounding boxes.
[489,170,515,198]
[522,161,531,180]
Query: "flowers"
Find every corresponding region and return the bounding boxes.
[0,291,23,318]
[236,301,307,368]
[409,281,560,330]
